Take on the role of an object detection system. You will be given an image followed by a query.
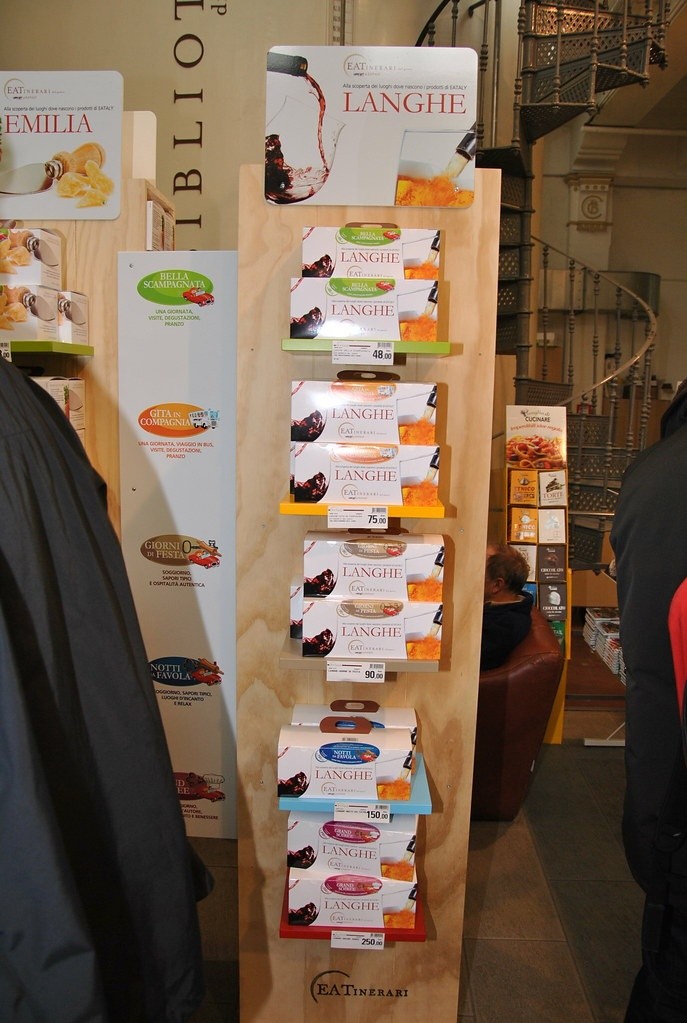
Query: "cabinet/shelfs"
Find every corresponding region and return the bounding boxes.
[508,465,571,746]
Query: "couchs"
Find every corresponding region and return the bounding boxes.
[470,606,564,819]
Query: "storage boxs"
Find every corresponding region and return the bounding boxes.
[290,525,445,660]
[287,811,417,929]
[277,700,418,801]
[290,222,441,341]
[0,228,90,449]
[290,370,440,507]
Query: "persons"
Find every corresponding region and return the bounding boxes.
[480,544,532,670]
[610,380,687,1023]
[0,355,214,1021]
